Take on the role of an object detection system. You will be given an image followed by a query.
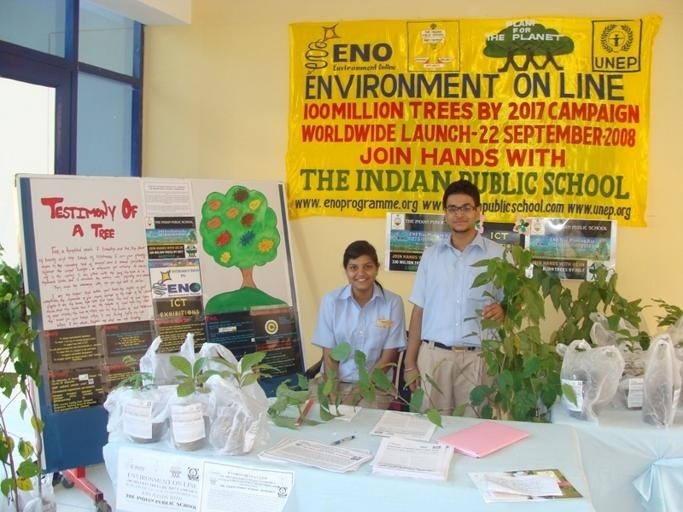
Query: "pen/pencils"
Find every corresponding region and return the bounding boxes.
[330,436,354,445]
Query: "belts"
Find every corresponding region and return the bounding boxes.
[424,339,476,351]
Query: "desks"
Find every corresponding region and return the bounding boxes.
[547,396,683,511]
[104,398,594,512]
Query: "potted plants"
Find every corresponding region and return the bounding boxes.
[103,332,281,455]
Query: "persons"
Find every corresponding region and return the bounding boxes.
[404,181,516,418]
[311,241,406,409]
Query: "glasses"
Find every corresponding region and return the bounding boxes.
[445,205,477,215]
